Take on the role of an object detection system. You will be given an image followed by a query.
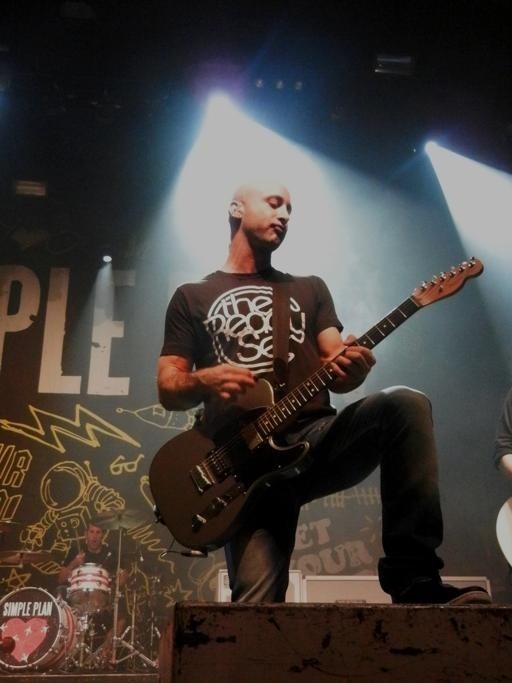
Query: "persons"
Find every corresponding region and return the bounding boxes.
[60,517,127,669]
[490,384,511,569]
[155,179,495,604]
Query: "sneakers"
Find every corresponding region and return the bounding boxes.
[392,575,491,610]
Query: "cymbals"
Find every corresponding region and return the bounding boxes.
[1,551,51,564]
[90,510,147,530]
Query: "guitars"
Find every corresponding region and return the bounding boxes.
[149,255,484,551]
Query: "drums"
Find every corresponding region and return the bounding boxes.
[0,586,76,672]
[69,562,112,614]
